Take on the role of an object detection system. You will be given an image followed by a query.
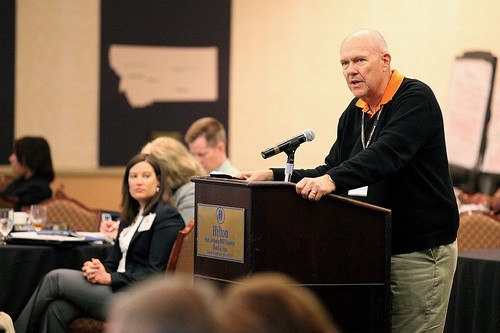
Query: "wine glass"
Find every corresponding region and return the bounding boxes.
[30,206,46,231]
[0,208,14,239]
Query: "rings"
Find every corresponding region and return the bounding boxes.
[311,191,316,194]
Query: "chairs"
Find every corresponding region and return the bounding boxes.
[458,211,500,250]
[23,190,103,233]
[68,220,197,333]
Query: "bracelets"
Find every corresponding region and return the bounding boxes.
[328,175,333,181]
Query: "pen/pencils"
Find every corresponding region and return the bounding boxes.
[108,219,121,232]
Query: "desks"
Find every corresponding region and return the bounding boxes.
[443,247,500,333]
[0,236,114,333]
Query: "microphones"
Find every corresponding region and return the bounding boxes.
[261,129,315,158]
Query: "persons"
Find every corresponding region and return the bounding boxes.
[218,272,344,333]
[0,136,54,212]
[14,153,187,333]
[140,136,208,228]
[235,28,459,333]
[184,116,242,177]
[102,272,235,333]
